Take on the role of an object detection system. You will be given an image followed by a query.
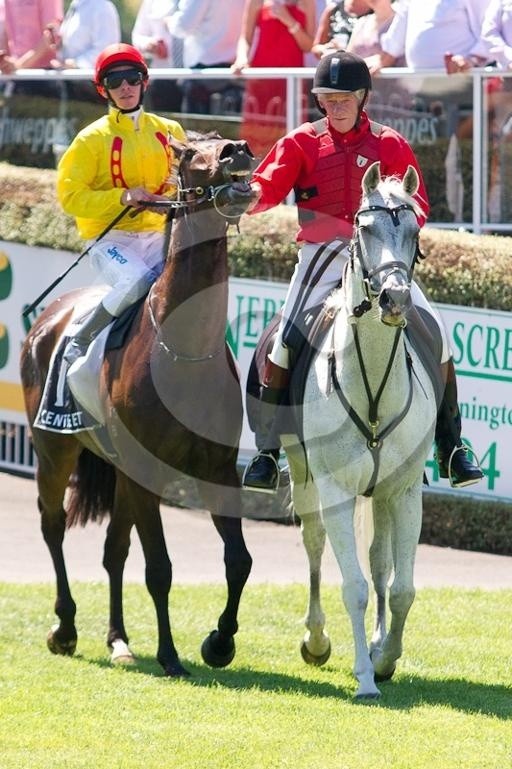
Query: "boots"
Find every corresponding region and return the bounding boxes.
[63,300,119,365]
[434,356,485,482]
[243,353,290,489]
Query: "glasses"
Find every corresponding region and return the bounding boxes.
[101,68,144,89]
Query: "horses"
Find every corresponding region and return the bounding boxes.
[276,160,438,710]
[19,129,256,680]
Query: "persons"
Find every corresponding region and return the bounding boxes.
[0,1,512,233]
[243,50,487,487]
[55,42,197,366]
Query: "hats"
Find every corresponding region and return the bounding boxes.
[91,43,148,99]
[310,48,373,95]
